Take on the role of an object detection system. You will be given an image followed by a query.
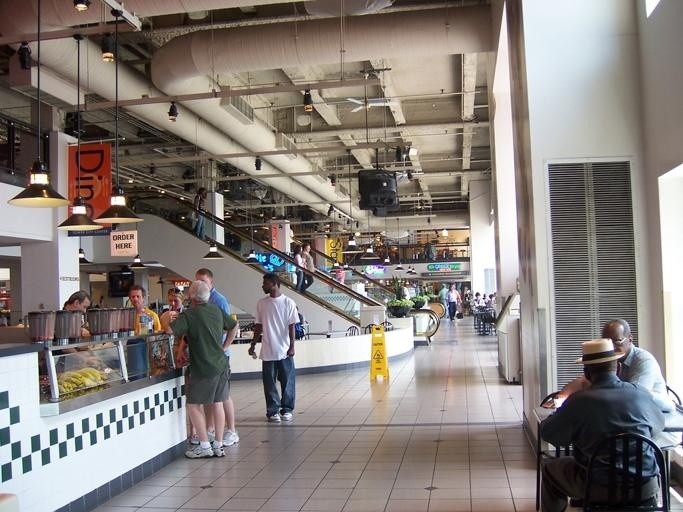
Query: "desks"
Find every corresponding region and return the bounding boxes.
[232,336,261,344]
[535,406,683,448]
[305,329,348,337]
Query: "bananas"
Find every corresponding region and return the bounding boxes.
[58,367,113,400]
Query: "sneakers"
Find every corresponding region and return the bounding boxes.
[269,414,281,422]
[223,429,240,446]
[206,431,215,442]
[282,413,292,421]
[185,441,214,458]
[189,434,200,445]
[212,441,226,457]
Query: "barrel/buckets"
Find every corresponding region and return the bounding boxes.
[88,308,102,334]
[121,309,129,331]
[69,310,81,338]
[129,308,135,330]
[23,311,43,341]
[44,311,55,340]
[55,310,69,338]
[113,309,121,333]
[102,309,113,333]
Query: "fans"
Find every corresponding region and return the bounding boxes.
[345,73,400,115]
[409,192,432,212]
[395,151,424,182]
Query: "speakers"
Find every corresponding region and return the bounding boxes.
[358,168,400,217]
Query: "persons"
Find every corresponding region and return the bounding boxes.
[161,288,187,314]
[301,242,315,291]
[128,285,161,337]
[494,292,497,296]
[463,294,470,301]
[195,268,240,447]
[539,337,666,512]
[194,187,208,242]
[543,318,678,413]
[170,280,238,458]
[293,246,307,288]
[434,283,451,318]
[445,286,462,322]
[483,293,487,299]
[51,290,91,356]
[488,294,494,301]
[468,294,475,300]
[248,273,300,422]
[474,293,486,307]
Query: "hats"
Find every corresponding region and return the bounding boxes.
[576,338,625,364]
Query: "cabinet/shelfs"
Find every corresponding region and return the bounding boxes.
[0,331,192,512]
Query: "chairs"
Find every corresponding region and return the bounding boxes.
[364,323,376,333]
[574,435,668,512]
[346,325,359,336]
[664,383,682,408]
[535,391,574,512]
[380,321,392,330]
[472,305,495,336]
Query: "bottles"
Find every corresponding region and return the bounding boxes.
[343,265,353,285]
[146,313,153,334]
[137,309,147,335]
[331,263,344,283]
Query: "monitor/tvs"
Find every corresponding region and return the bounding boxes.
[109,271,135,297]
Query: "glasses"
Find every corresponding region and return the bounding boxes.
[614,338,626,344]
[168,289,181,295]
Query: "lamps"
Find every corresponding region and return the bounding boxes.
[360,245,379,259]
[100,29,115,62]
[442,228,448,237]
[407,267,411,274]
[7,159,72,208]
[331,172,335,186]
[341,239,364,254]
[411,267,418,275]
[16,40,33,69]
[130,255,146,270]
[93,186,142,224]
[331,262,341,270]
[203,243,224,260]
[245,252,261,264]
[79,248,89,264]
[303,89,313,111]
[255,155,262,170]
[343,262,354,272]
[167,101,179,122]
[55,195,103,230]
[395,265,404,271]
[73,0,91,12]
[382,255,390,265]
